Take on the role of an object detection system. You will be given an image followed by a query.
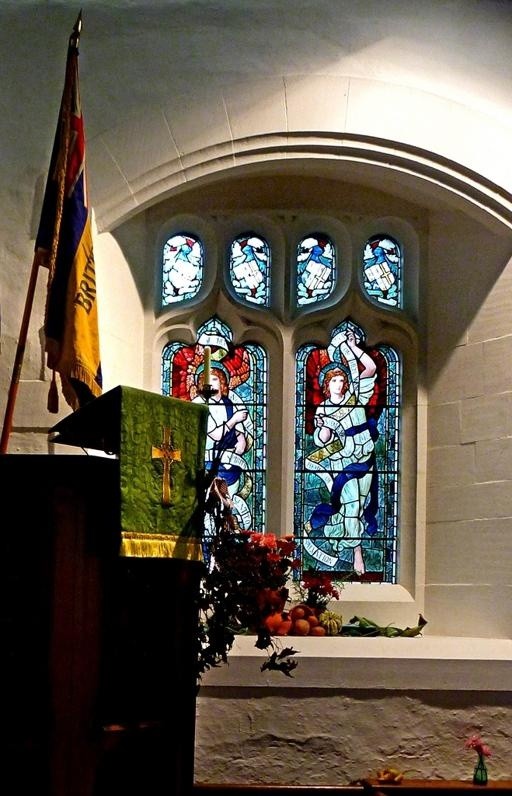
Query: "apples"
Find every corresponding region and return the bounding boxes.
[291,608,326,636]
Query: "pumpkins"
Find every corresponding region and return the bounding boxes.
[263,612,291,635]
[320,611,343,635]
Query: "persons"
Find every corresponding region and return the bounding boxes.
[190,358,251,577]
[311,327,379,578]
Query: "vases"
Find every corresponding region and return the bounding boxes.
[300,600,327,620]
[472,758,487,783]
[245,586,286,623]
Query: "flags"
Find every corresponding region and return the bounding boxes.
[32,42,103,413]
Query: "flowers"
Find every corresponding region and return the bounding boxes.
[239,529,296,596]
[296,564,340,606]
[463,734,492,762]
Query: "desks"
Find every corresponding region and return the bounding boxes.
[359,779,512,796]
